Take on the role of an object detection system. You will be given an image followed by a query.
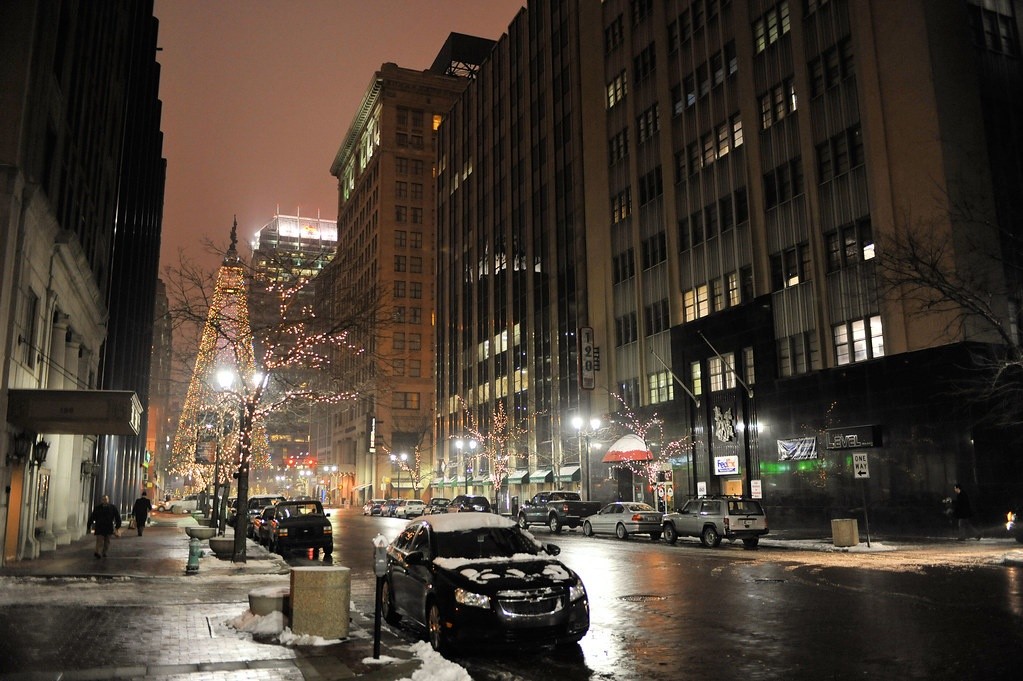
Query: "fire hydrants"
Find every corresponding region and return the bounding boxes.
[186,537,205,575]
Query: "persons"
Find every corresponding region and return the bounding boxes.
[86,495,121,559]
[131,492,152,536]
[942,484,982,542]
[713,407,733,441]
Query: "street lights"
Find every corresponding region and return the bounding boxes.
[276,475,285,498]
[299,470,312,499]
[572,415,603,501]
[390,453,408,499]
[323,465,338,506]
[455,439,478,493]
[217,366,270,536]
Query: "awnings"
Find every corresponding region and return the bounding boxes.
[354,483,372,490]
[602,434,653,463]
[391,482,424,488]
[429,466,581,487]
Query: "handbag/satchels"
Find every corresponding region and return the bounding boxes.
[115,529,121,537]
[128,519,137,529]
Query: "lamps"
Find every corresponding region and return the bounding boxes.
[4,431,32,467]
[90,462,100,478]
[28,437,49,470]
[81,458,92,478]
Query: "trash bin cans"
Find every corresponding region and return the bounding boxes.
[831,518,859,547]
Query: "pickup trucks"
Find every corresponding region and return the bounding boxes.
[517,490,601,533]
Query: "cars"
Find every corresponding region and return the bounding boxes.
[270,500,334,554]
[219,496,287,544]
[660,496,769,548]
[368,512,591,663]
[166,494,221,514]
[581,501,663,539]
[361,495,490,520]
